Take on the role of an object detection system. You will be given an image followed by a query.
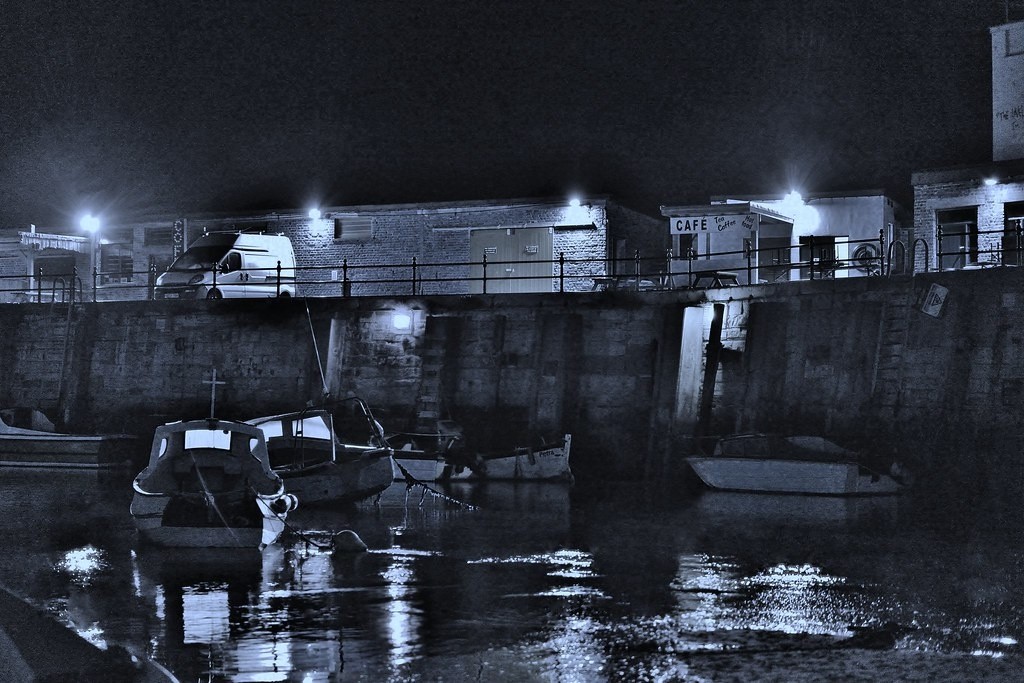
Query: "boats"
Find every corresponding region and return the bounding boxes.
[680,433,904,495]
[128,369,298,547]
[373,420,486,481]
[247,393,396,509]
[466,431,592,482]
[0,404,137,452]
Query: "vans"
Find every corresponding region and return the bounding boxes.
[152,228,296,298]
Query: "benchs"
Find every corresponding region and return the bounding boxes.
[10,292,57,302]
[589,277,618,292]
[815,262,892,280]
[692,270,740,287]
